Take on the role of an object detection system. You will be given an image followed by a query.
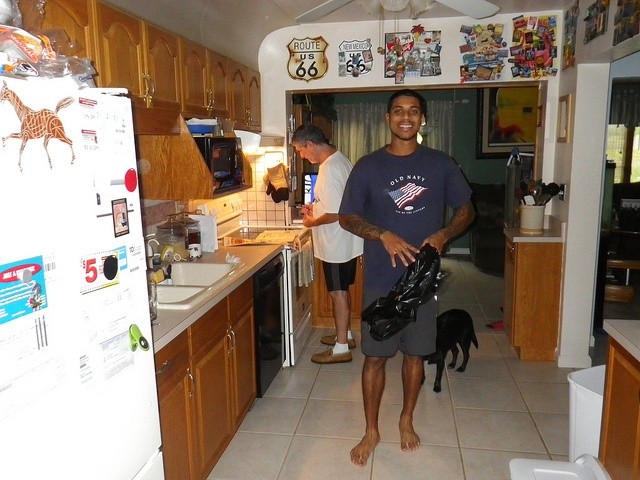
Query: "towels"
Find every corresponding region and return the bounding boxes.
[298,241,316,288]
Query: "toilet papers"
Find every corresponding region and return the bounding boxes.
[188,214,214,252]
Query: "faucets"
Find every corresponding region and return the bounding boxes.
[142,234,160,269]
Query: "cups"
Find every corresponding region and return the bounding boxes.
[188,243,202,260]
[518,206,545,235]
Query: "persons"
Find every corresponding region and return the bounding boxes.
[338,89,475,467]
[291,124,364,364]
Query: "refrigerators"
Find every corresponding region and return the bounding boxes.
[0,76,167,480]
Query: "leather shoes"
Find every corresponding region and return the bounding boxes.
[320,335,356,349]
[311,349,352,364]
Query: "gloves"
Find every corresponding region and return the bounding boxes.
[263,162,289,187]
[265,165,289,203]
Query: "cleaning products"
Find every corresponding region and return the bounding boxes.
[161,238,176,263]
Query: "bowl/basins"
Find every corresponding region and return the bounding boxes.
[186,123,219,136]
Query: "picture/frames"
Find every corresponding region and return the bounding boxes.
[473,89,547,158]
[554,97,571,147]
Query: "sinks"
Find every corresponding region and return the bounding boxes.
[152,287,206,303]
[163,263,234,287]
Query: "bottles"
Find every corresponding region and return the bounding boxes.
[179,215,205,244]
[156,215,186,247]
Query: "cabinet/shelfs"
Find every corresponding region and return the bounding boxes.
[256,250,284,400]
[195,283,258,475]
[182,31,231,123]
[5,0,95,91]
[154,323,196,474]
[234,56,263,134]
[318,246,362,329]
[597,318,640,475]
[501,231,560,364]
[99,2,179,134]
[285,249,316,364]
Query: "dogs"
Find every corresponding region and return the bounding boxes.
[420,309,478,393]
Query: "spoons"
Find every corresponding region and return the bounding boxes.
[530,185,539,205]
[542,179,560,205]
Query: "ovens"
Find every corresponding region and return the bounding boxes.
[193,138,244,195]
